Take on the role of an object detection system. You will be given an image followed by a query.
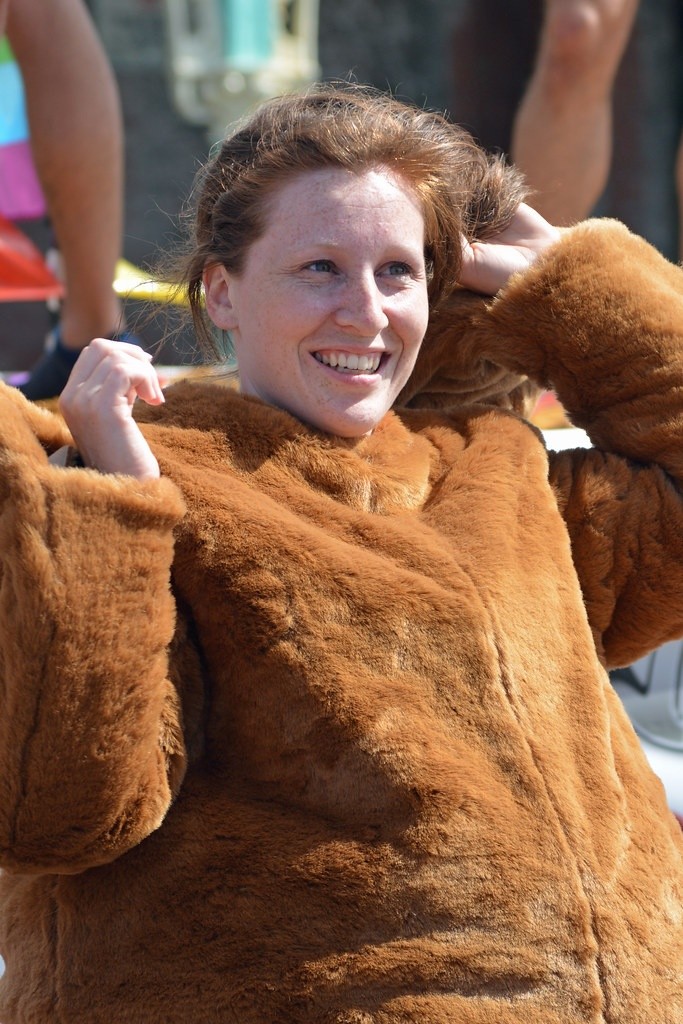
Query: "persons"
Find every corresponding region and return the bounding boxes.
[0,89,683,1024]
[510,0,683,428]
[0,0,145,401]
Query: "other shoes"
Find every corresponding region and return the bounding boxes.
[19,324,147,404]
